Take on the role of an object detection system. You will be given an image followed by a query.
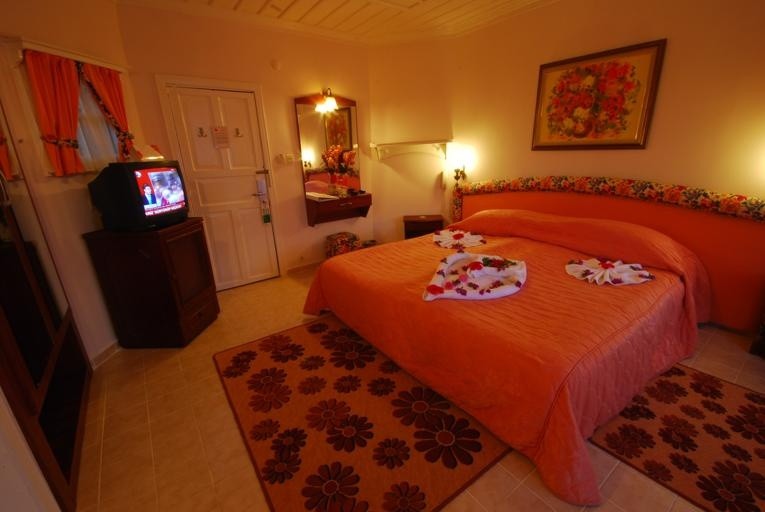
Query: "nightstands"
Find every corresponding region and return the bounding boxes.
[402,214,446,240]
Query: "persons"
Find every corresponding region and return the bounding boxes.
[142,176,186,209]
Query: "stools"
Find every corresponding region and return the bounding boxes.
[322,232,362,261]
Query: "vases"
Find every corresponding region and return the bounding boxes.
[337,168,351,196]
[328,168,338,196]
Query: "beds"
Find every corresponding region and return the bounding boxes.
[304,174,765,511]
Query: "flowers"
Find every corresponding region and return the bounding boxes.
[321,145,343,180]
[340,149,357,173]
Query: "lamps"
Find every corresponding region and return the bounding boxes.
[444,141,468,182]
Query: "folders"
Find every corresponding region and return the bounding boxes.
[306,191,340,203]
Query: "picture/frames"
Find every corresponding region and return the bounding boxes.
[322,107,354,154]
[529,36,669,152]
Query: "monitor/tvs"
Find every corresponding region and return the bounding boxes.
[88,159,189,232]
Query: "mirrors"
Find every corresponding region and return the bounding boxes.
[293,88,374,194]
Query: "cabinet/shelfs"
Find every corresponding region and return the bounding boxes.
[304,192,374,227]
[78,215,222,350]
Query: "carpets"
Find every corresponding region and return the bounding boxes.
[211,310,515,512]
[587,358,765,512]
